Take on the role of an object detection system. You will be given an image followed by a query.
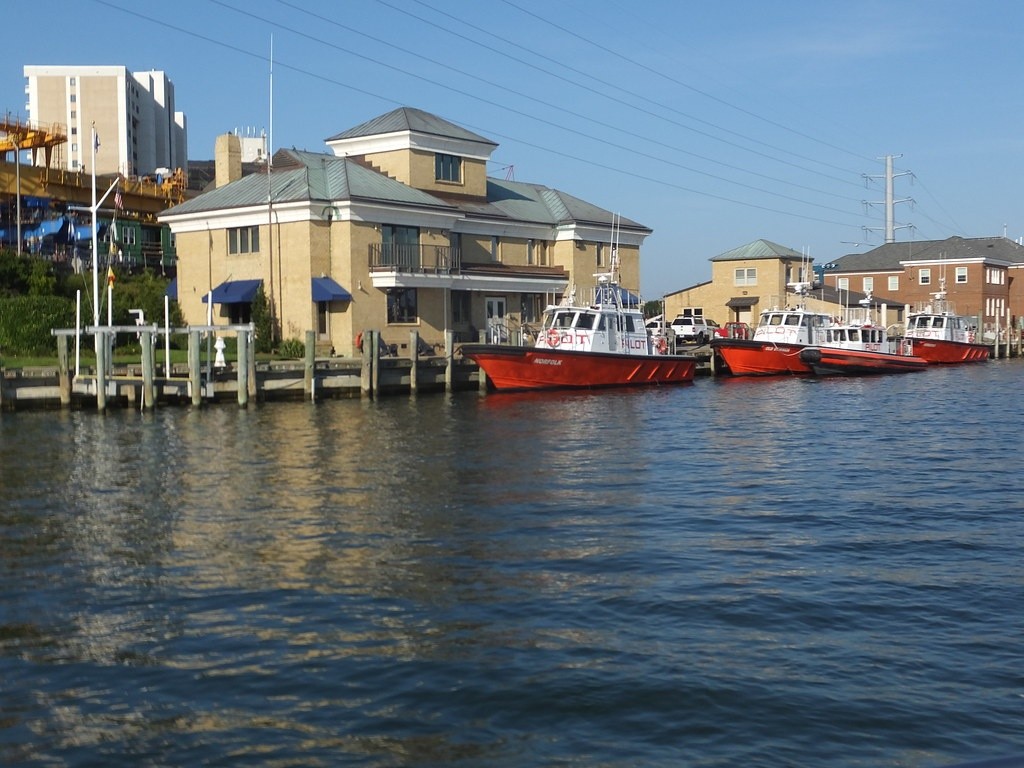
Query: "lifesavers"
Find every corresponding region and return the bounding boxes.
[547,329,560,346]
[657,338,667,353]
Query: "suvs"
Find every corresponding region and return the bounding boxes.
[671,314,722,346]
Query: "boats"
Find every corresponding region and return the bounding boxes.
[888,253,994,366]
[800,290,929,378]
[451,212,699,390]
[709,246,846,378]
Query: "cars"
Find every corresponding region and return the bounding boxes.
[715,321,751,341]
[645,320,671,336]
